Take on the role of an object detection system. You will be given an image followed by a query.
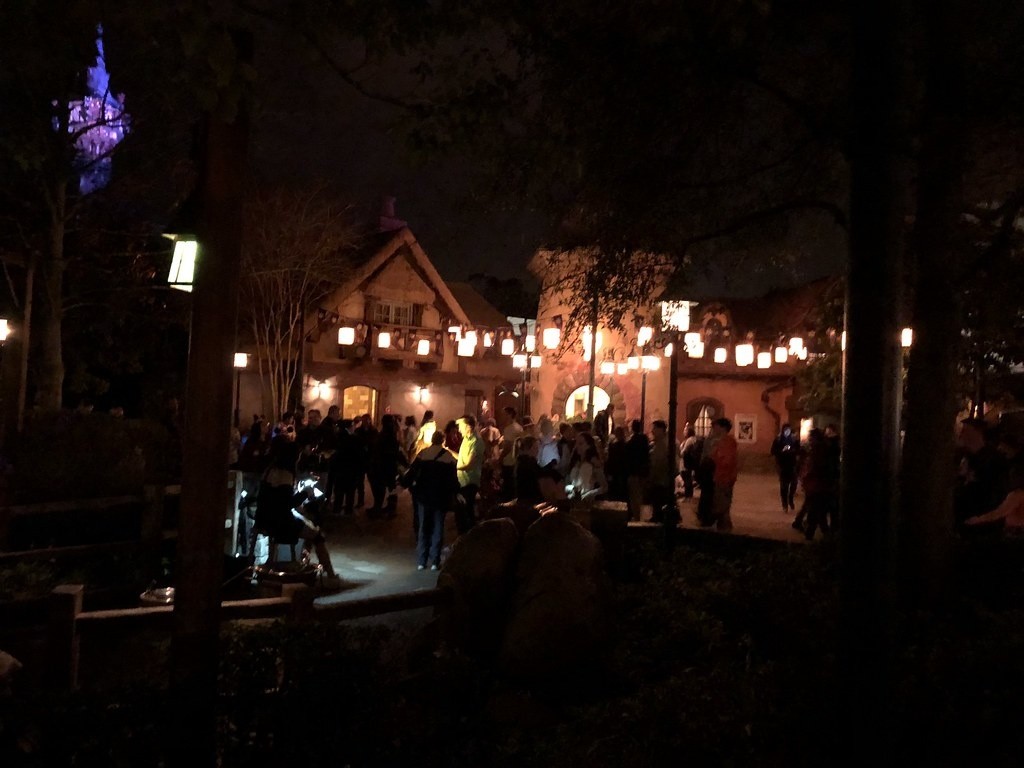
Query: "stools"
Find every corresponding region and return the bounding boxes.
[249,528,297,563]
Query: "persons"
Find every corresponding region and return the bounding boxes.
[956,417,1024,546]
[249,407,435,516]
[648,419,668,520]
[76,397,182,419]
[401,432,462,570]
[568,434,609,511]
[255,442,341,579]
[446,420,462,453]
[675,436,681,522]
[825,425,841,464]
[609,425,630,501]
[536,472,569,516]
[445,415,482,534]
[628,419,646,521]
[481,404,615,485]
[681,424,696,502]
[490,471,543,534]
[802,430,834,538]
[793,427,821,528]
[699,419,738,532]
[229,425,255,471]
[773,425,800,513]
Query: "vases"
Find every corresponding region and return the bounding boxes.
[264,563,319,597]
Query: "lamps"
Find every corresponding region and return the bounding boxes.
[145,235,197,294]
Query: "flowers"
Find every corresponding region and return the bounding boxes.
[255,550,323,579]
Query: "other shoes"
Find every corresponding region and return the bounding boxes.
[430,565,440,572]
[331,500,397,519]
[791,518,829,542]
[782,499,795,513]
[418,564,427,571]
